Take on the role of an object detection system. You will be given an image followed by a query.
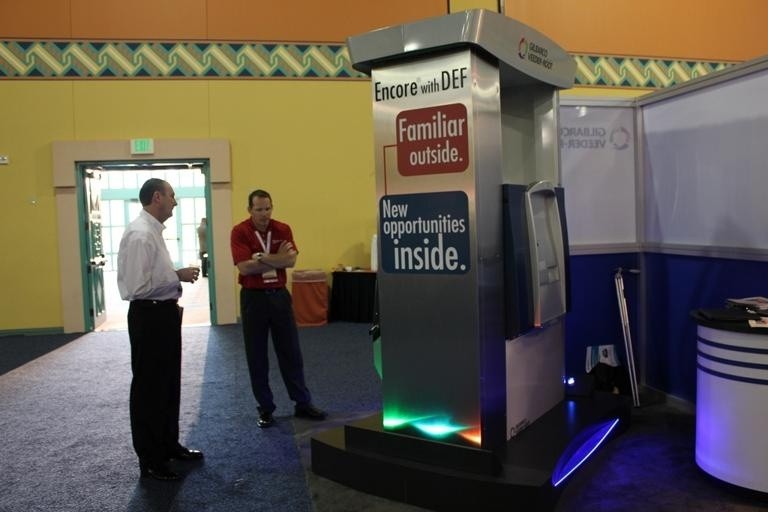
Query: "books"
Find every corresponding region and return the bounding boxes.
[729,296,768,310]
[748,316,768,328]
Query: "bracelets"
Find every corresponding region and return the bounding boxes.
[256,252,263,259]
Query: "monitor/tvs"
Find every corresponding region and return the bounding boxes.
[530,195,556,257]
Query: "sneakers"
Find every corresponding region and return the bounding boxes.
[166,448,203,465]
[293,402,329,421]
[138,463,181,482]
[255,412,274,428]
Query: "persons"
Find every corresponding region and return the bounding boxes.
[230,189,328,428]
[197,217,209,278]
[117,178,203,481]
